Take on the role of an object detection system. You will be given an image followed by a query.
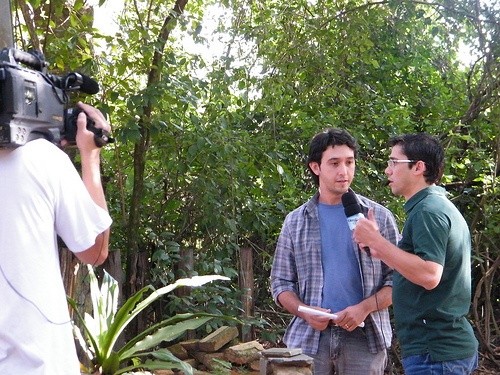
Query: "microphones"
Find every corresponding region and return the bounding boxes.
[75,71,99,95]
[341,192,372,258]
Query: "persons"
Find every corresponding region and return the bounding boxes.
[270,128,398,375]
[353,133,480,375]
[0,101,114,375]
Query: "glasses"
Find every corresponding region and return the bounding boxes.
[387,158,426,168]
